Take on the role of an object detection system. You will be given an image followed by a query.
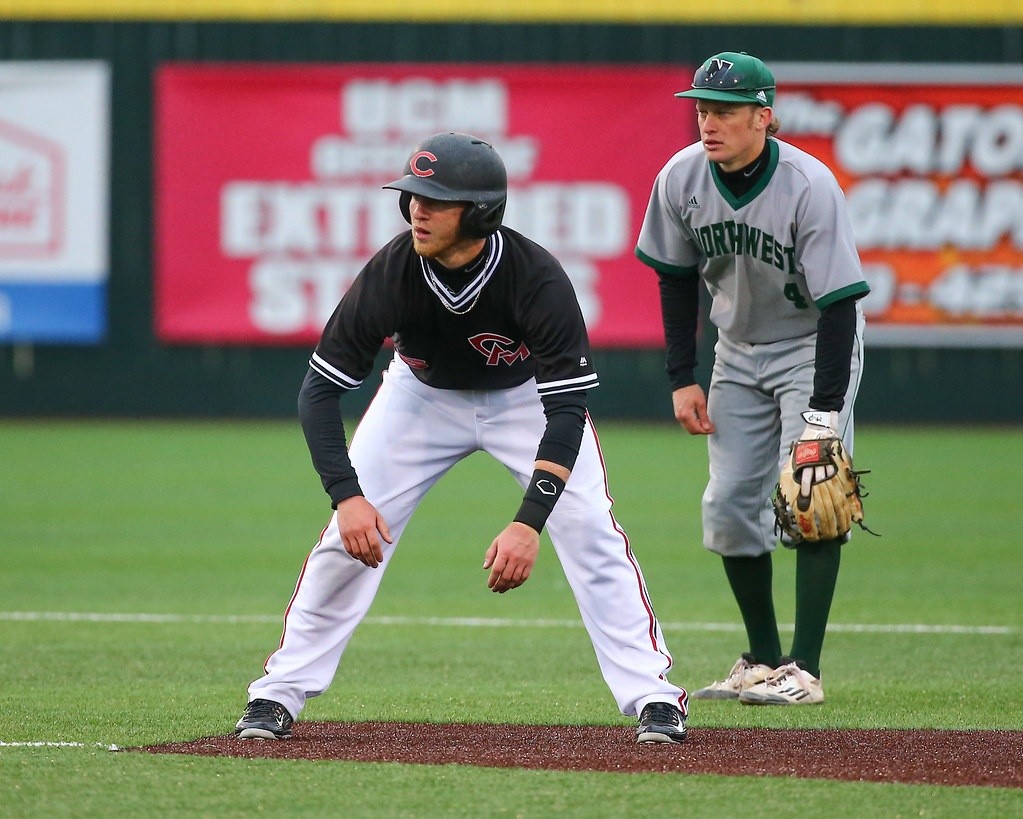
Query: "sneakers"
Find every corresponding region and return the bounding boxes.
[637,702,687,745]
[236,698,292,740]
[739,660,824,705]
[692,659,776,699]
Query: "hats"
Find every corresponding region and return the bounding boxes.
[673,52,775,109]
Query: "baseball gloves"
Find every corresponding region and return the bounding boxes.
[772,430,861,544]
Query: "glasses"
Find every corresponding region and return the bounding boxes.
[691,66,774,90]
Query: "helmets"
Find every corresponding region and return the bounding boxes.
[382,132,508,237]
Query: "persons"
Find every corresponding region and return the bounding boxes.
[234,133,689,744]
[634,51,871,706]
